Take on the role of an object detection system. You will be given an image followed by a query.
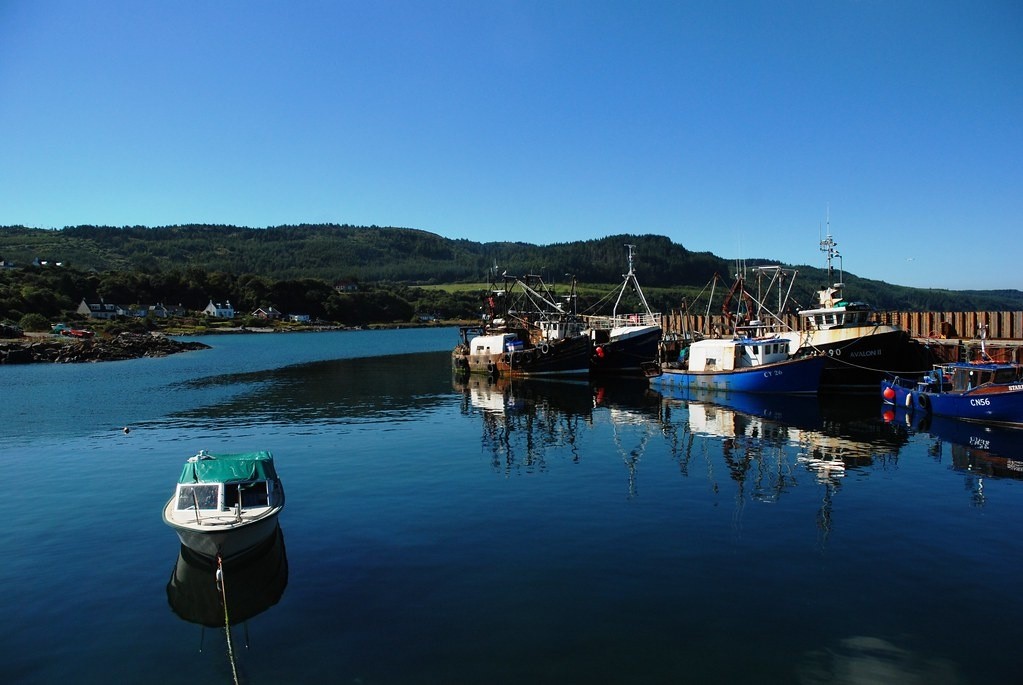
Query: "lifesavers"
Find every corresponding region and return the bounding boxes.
[824,299,833,307]
[487,364,495,373]
[918,417,929,432]
[918,394,930,409]
[968,360,988,365]
[504,341,549,365]
[487,375,495,386]
[752,337,764,340]
[989,360,1010,364]
[455,357,468,368]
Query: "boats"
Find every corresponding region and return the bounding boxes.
[644,380,910,556]
[449,243,664,384]
[168,517,289,655]
[639,201,922,398]
[880,320,1023,429]
[877,400,1023,519]
[161,448,286,565]
